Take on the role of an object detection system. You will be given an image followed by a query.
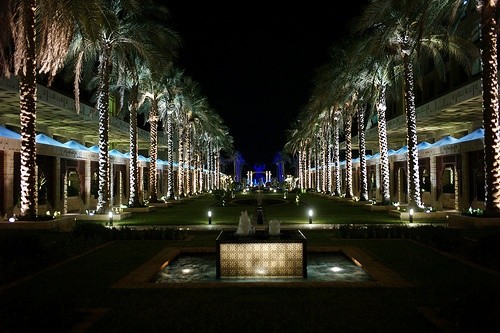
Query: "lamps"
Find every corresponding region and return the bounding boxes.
[309,210,313,224]
[409,209,413,223]
[109,212,114,226]
[208,211,212,224]
